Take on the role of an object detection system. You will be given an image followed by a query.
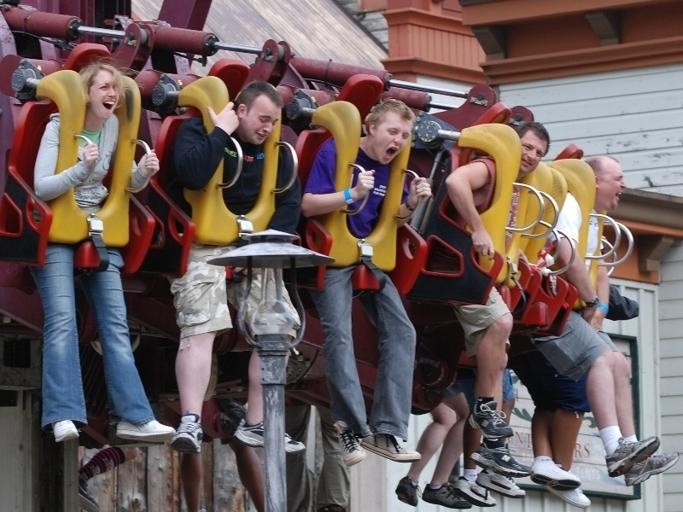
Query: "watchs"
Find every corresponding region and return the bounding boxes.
[585,294,599,308]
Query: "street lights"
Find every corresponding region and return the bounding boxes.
[210,228,337,511]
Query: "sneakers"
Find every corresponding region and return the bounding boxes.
[360,432,423,463]
[623,451,680,488]
[470,443,534,476]
[395,478,419,507]
[604,434,660,479]
[231,416,308,455]
[333,419,366,468]
[170,413,205,455]
[475,467,527,497]
[422,472,498,508]
[468,400,514,439]
[50,418,81,444]
[77,483,101,511]
[530,459,582,492]
[115,416,176,442]
[543,484,588,510]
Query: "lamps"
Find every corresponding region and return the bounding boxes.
[586,8,622,43]
[472,26,504,54]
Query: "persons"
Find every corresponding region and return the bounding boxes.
[442,120,550,479]
[178,434,263,511]
[167,80,307,455]
[284,391,351,511]
[508,338,591,508]
[77,446,139,511]
[299,96,434,465]
[25,60,179,445]
[450,349,527,508]
[393,384,472,512]
[529,155,680,487]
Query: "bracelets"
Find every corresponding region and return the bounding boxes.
[342,185,353,205]
[598,302,609,314]
[403,199,416,212]
